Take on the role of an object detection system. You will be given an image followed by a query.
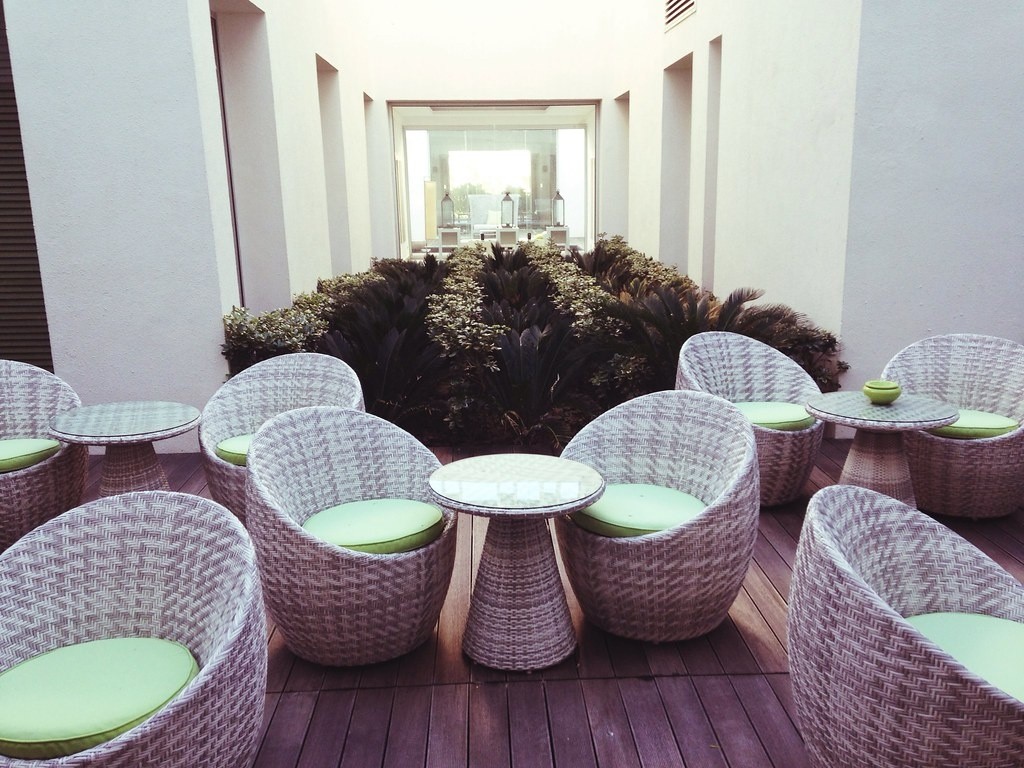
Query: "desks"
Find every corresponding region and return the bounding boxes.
[48,401,201,500]
[428,453,606,672]
[806,391,960,508]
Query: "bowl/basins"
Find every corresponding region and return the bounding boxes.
[863,381,901,404]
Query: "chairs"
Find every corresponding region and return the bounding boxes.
[197,353,366,528]
[786,484,1024,768]
[0,491,268,768]
[555,389,761,643]
[882,333,1024,516]
[675,330,824,507]
[0,359,90,552]
[244,405,458,667]
